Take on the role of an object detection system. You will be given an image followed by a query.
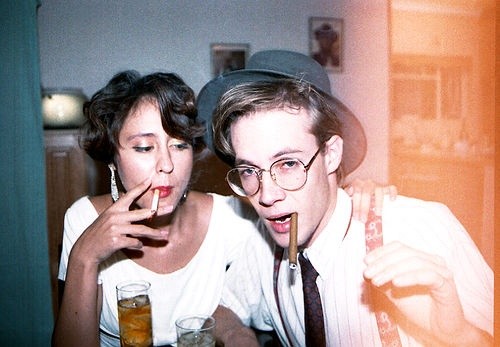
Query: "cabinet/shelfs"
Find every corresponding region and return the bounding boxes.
[43,130,242,321]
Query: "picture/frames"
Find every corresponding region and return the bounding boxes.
[209,44,249,77]
[307,17,344,73]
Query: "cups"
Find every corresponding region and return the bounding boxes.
[115,280,153,347]
[175,314,216,347]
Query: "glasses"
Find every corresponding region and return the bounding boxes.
[224,142,324,198]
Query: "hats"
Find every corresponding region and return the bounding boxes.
[195,50,367,178]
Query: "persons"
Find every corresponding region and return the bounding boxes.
[50,68,398,347]
[194,50,494,347]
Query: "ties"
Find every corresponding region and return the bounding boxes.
[298,249,326,347]
